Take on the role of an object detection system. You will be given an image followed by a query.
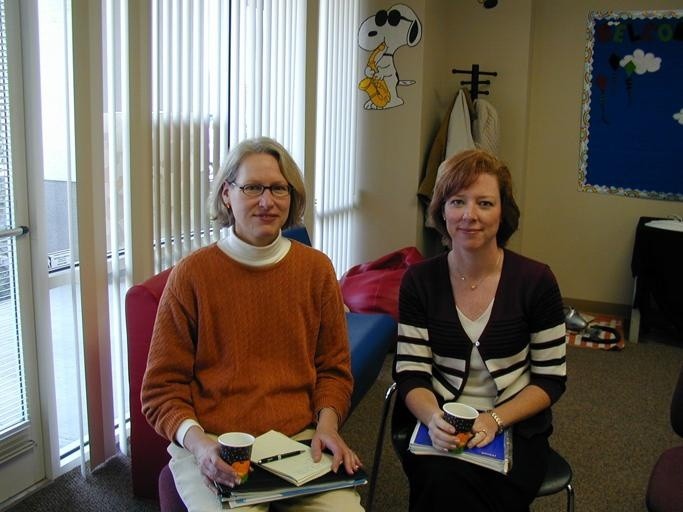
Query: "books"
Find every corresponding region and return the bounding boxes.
[215,429,368,509]
[408,418,514,475]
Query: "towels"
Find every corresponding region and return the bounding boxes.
[416,88,498,228]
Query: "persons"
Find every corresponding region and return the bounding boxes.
[140,136,365,512]
[392,149,568,512]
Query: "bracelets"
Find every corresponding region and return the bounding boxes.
[487,409,504,436]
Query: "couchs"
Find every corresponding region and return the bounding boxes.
[125,265,173,500]
[279,226,397,432]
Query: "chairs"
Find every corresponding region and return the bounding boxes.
[646,371,683,512]
[158,464,188,512]
[364,381,574,512]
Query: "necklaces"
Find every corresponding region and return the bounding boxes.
[451,250,501,290]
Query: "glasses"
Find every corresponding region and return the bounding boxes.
[231,182,294,199]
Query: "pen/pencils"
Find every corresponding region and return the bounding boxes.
[257,450,306,467]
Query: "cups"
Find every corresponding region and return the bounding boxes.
[436,401,480,450]
[216,430,254,485]
